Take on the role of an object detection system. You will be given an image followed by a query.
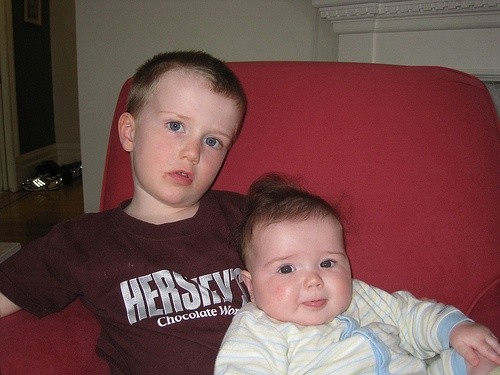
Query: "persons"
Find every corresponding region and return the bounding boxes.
[211,171,500,375]
[0,49,252,375]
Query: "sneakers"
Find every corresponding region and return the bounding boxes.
[21,174,63,190]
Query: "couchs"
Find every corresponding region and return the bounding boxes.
[0,61,500,375]
